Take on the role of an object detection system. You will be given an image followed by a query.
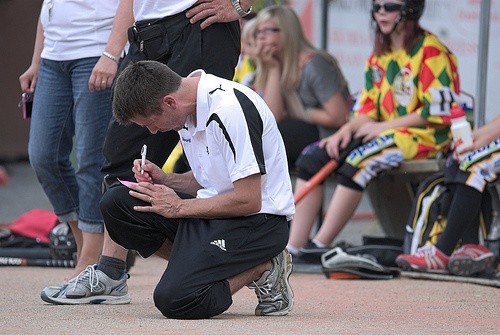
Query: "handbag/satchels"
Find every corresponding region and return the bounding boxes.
[11,208,59,243]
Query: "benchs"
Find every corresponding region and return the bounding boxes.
[318,89,474,241]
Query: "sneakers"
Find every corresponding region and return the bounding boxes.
[444,244,495,277]
[396,242,449,274]
[40,264,131,306]
[247,247,295,316]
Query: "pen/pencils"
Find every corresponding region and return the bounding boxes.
[138,142,149,176]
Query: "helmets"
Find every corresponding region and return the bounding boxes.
[370,1,425,20]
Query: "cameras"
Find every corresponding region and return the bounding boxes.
[22,93,33,119]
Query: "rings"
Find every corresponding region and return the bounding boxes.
[101,81,107,84]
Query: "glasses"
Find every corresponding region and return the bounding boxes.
[254,27,281,35]
[372,2,403,12]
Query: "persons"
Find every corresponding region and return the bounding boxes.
[98,60,296,320]
[285,0,461,264]
[19,0,136,283]
[162,6,351,243]
[41,0,255,305]
[395,116,500,276]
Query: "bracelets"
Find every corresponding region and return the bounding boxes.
[103,52,119,64]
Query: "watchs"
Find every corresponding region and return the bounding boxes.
[230,0,252,18]
